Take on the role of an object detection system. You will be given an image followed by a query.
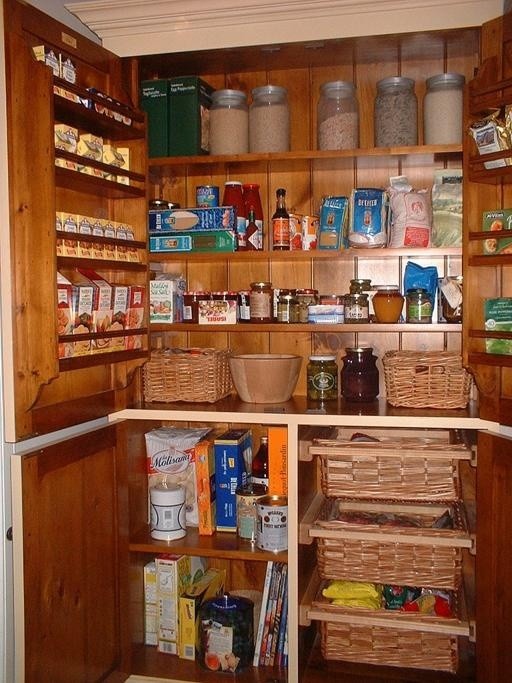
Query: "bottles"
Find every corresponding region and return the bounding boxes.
[222,179,262,251]
[183,276,460,325]
[340,346,379,403]
[207,70,465,154]
[269,186,291,250]
[253,435,268,486]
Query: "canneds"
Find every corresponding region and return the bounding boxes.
[248,282,274,324]
[408,293,432,323]
[236,483,268,542]
[277,295,300,324]
[343,294,370,324]
[349,279,371,293]
[255,495,288,553]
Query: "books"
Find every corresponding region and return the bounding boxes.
[254,561,288,670]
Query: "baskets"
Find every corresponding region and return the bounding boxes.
[382,350,473,410]
[142,347,238,403]
[314,500,461,592]
[312,580,460,674]
[313,426,468,507]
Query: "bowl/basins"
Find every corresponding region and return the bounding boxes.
[226,352,303,405]
